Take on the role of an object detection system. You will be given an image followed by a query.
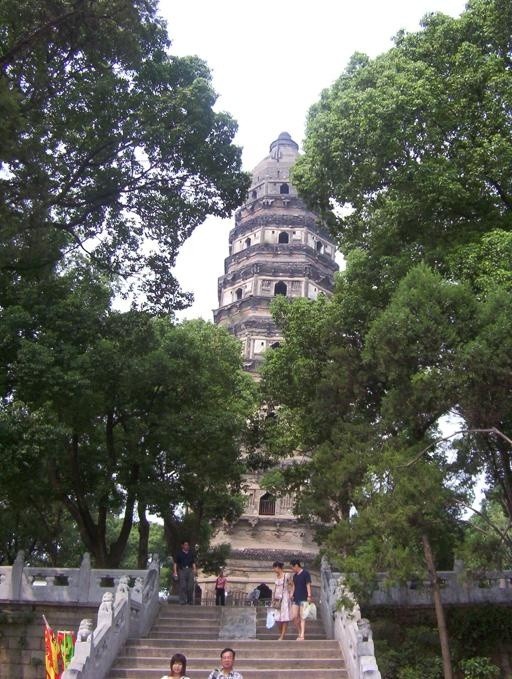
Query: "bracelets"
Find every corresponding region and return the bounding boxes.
[308,596,312,598]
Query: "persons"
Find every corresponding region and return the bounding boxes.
[215,571,226,606]
[248,586,262,606]
[160,653,191,679]
[207,648,244,679]
[290,559,312,640]
[173,540,197,604]
[269,561,295,640]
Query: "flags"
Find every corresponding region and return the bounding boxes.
[44,625,61,679]
[57,631,76,672]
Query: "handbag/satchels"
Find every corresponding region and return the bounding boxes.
[271,598,282,609]
